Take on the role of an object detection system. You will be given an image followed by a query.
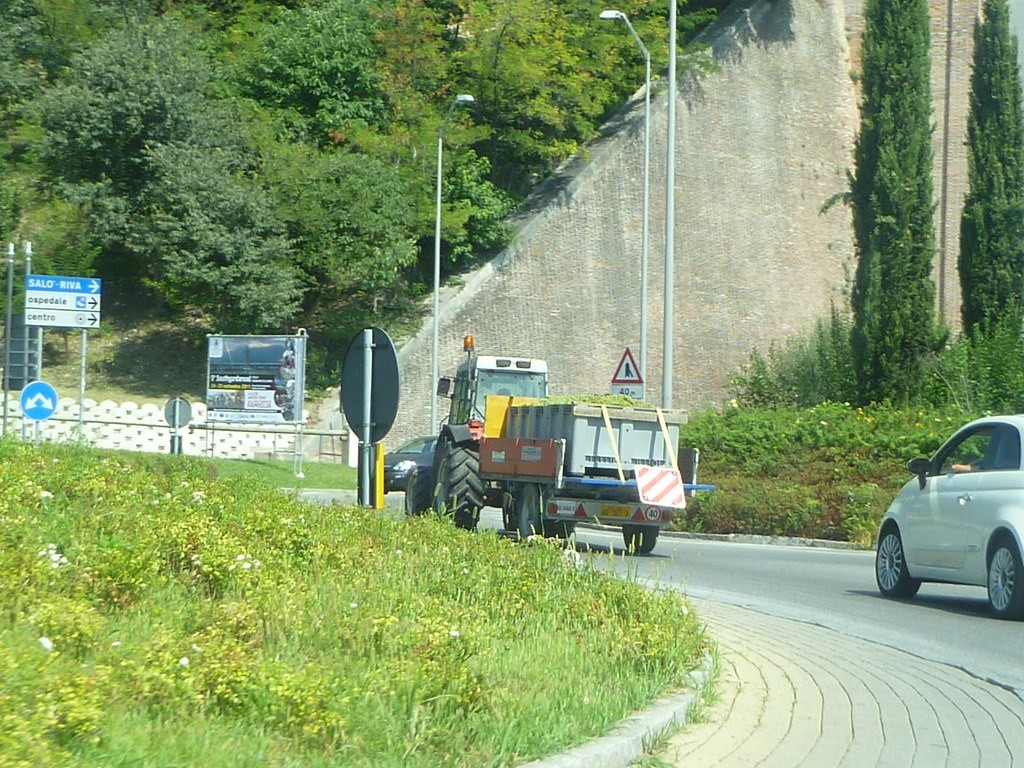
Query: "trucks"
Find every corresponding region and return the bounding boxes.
[401,335,701,558]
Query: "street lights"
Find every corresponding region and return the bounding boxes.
[430,93,476,430]
[600,8,651,402]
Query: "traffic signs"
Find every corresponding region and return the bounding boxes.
[24,276,100,327]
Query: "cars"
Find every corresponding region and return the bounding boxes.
[871,413,1024,624]
[380,434,440,492]
